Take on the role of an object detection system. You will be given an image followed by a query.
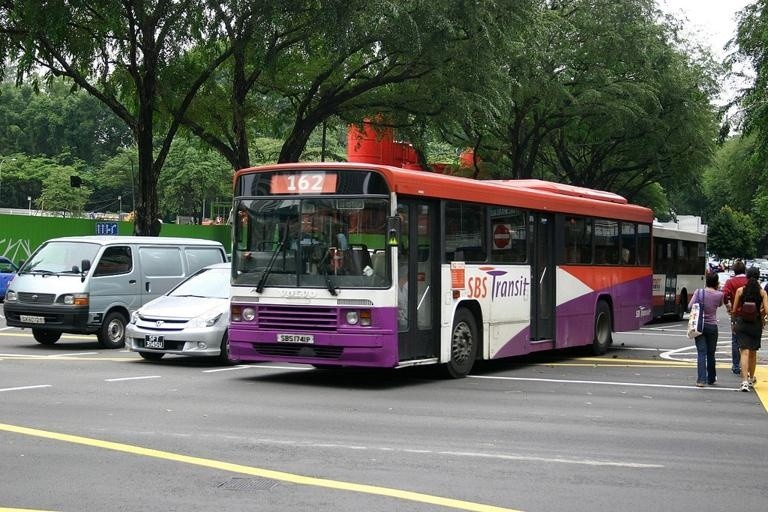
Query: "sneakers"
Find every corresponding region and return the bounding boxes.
[739,376,756,392]
[696,382,704,387]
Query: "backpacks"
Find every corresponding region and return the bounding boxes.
[742,301,757,321]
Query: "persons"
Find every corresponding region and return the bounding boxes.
[722,260,768,391]
[688,272,724,387]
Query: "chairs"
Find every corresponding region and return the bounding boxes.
[347,243,429,276]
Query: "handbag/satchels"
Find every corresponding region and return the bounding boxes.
[686,303,705,339]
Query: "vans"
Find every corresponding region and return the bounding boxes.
[4,235,226,347]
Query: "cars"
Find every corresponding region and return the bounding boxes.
[709,256,768,281]
[123,262,241,365]
